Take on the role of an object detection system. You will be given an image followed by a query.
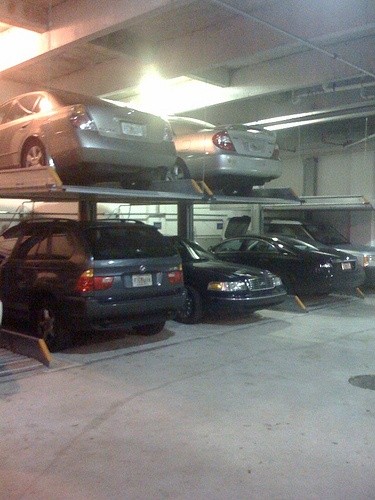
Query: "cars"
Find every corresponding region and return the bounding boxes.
[164,236,288,324]
[207,236,364,294]
[0,90,178,190]
[165,116,282,197]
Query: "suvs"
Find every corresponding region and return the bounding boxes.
[0,218,188,351]
[222,216,375,276]
